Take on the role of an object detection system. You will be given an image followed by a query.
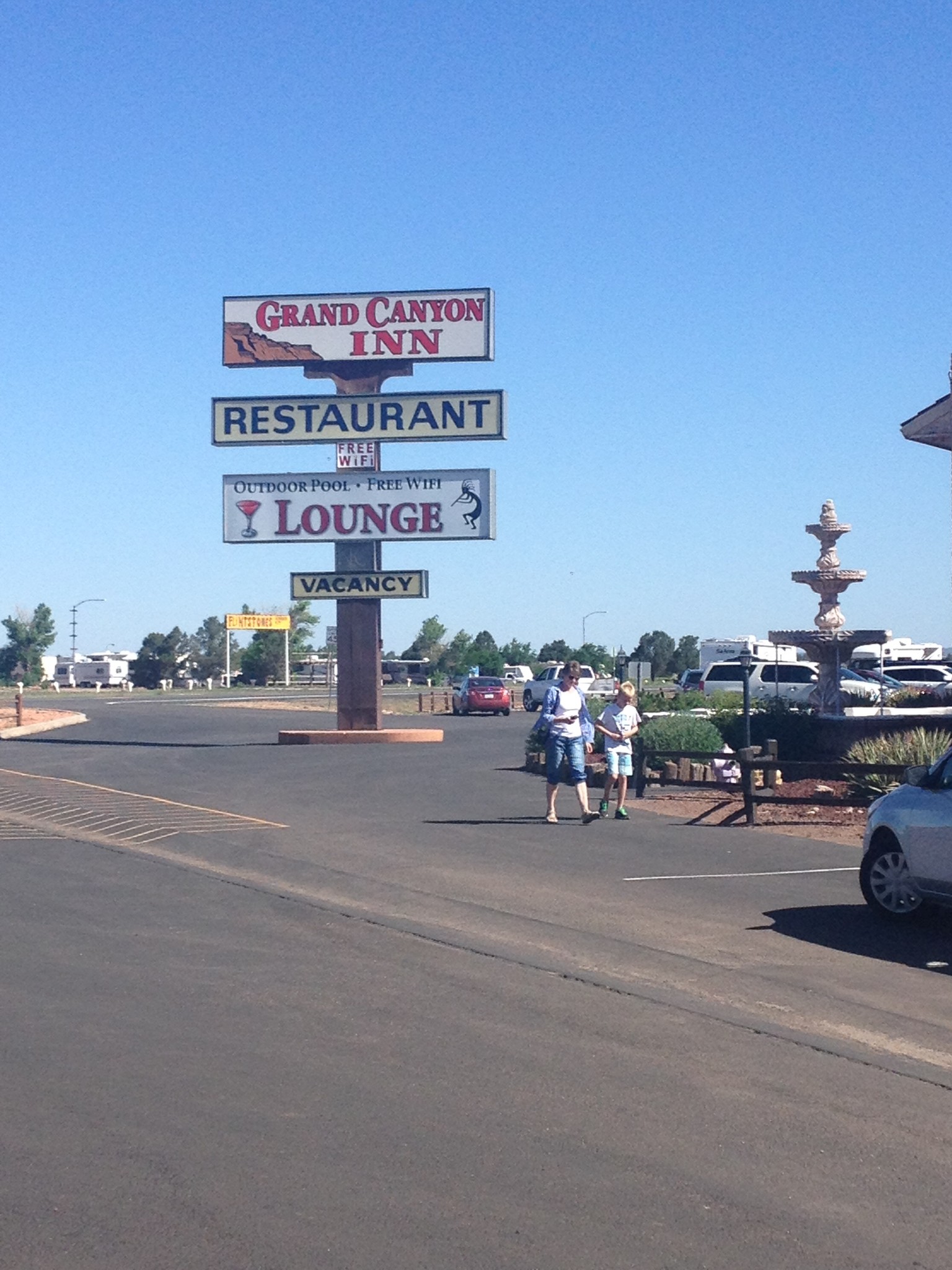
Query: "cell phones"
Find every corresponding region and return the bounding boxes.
[570,715,577,720]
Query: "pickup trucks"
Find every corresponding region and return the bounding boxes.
[523,666,620,711]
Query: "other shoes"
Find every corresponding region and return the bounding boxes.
[546,812,558,823]
[599,809,609,817]
[615,811,630,820]
[581,811,600,824]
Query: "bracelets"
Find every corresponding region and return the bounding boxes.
[610,733,614,737]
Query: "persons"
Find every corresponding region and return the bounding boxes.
[594,680,643,821]
[528,659,601,825]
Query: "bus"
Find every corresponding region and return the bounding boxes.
[289,656,338,684]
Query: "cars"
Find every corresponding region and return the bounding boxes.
[681,662,952,701]
[451,677,509,716]
[858,742,952,920]
[381,659,533,684]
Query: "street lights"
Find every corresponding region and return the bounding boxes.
[582,612,608,645]
[69,599,106,663]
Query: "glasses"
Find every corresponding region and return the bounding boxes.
[568,675,579,681]
